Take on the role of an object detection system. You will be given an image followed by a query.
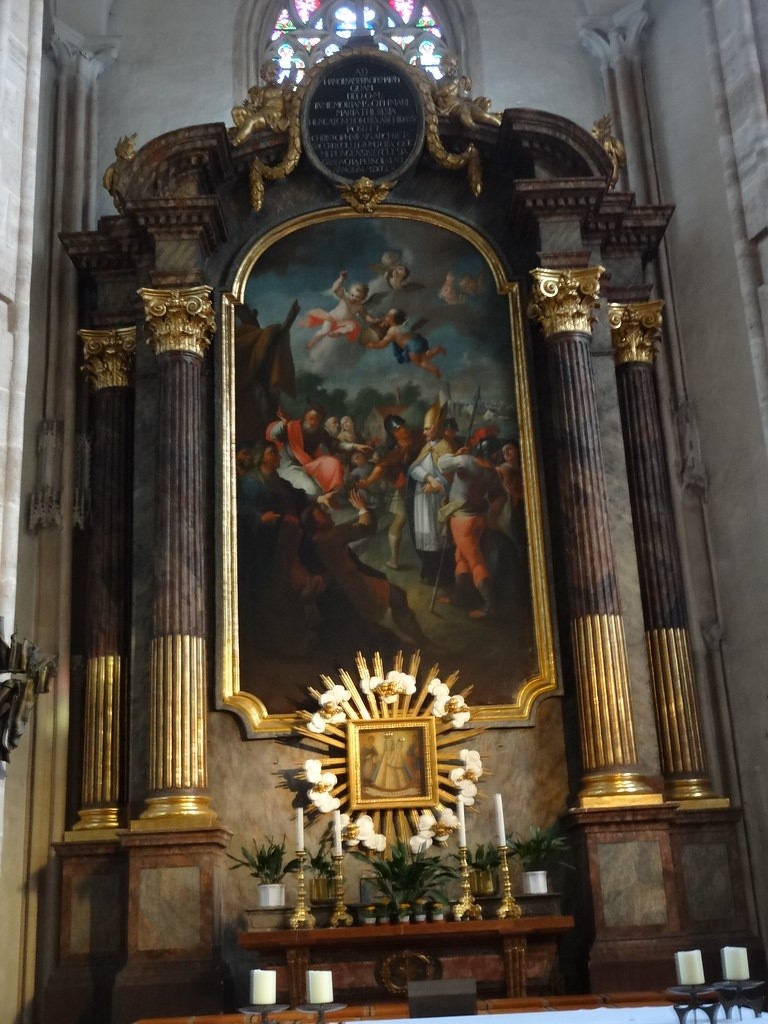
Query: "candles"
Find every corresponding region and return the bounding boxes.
[296,807,304,851]
[719,946,749,981]
[332,810,342,856]
[249,969,277,1005]
[494,793,506,846]
[305,971,334,1004]
[456,795,466,847]
[675,949,704,984]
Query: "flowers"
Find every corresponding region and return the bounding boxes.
[415,898,428,915]
[380,897,392,917]
[432,903,443,915]
[399,903,411,919]
[365,906,376,918]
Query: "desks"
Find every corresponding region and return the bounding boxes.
[236,915,583,1009]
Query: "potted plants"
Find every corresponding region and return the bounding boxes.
[224,834,306,908]
[447,843,503,896]
[303,822,334,905]
[505,822,574,896]
[348,836,472,924]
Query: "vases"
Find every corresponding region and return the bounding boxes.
[415,915,426,921]
[433,914,443,920]
[399,916,410,922]
[379,918,390,923]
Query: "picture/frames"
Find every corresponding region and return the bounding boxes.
[347,714,441,810]
[365,918,376,924]
